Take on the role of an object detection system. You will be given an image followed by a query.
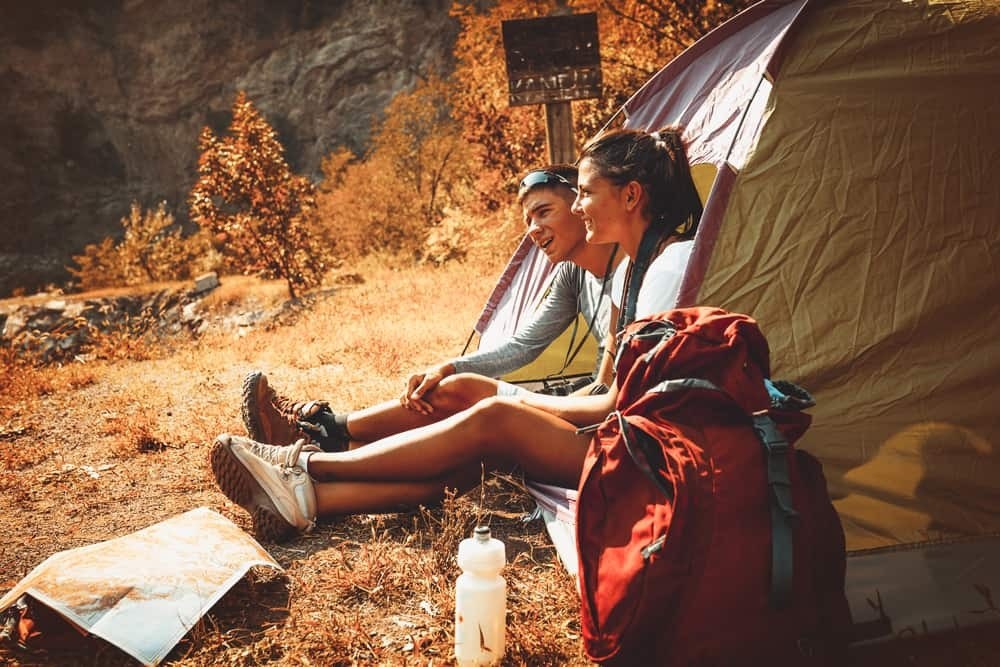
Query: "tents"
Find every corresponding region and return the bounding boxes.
[461,0,1000,648]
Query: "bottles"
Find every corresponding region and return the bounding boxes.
[456,527,507,667]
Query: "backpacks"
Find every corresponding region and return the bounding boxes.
[572,305,854,667]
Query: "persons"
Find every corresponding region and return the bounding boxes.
[240,164,629,451]
[209,125,704,545]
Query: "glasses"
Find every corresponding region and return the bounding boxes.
[518,170,579,203]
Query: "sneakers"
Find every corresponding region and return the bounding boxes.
[210,433,318,545]
[236,435,326,484]
[269,385,333,421]
[239,372,335,446]
